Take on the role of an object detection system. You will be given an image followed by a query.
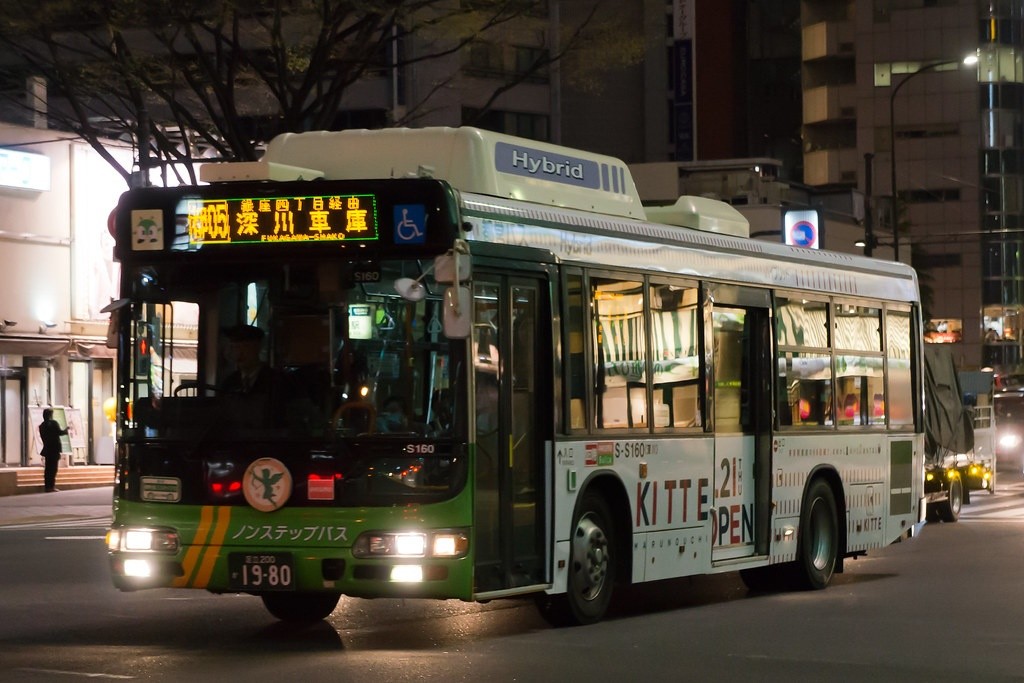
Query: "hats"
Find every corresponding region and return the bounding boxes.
[218,324,265,339]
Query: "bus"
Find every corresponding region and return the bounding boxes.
[99,125,929,623]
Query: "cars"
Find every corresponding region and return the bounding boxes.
[994,370,1024,472]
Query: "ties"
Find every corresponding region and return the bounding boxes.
[239,378,250,393]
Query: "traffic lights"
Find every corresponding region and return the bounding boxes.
[139,339,150,374]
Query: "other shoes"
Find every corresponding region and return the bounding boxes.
[52,488,59,492]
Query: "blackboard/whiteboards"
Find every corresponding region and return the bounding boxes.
[45,406,73,456]
[28,405,50,456]
[64,407,87,448]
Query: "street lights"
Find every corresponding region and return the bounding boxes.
[887,53,981,266]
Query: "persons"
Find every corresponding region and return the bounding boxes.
[512,311,566,503]
[217,327,280,407]
[39,409,70,492]
[384,397,432,437]
[430,387,455,434]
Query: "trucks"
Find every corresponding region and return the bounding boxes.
[923,407,997,525]
